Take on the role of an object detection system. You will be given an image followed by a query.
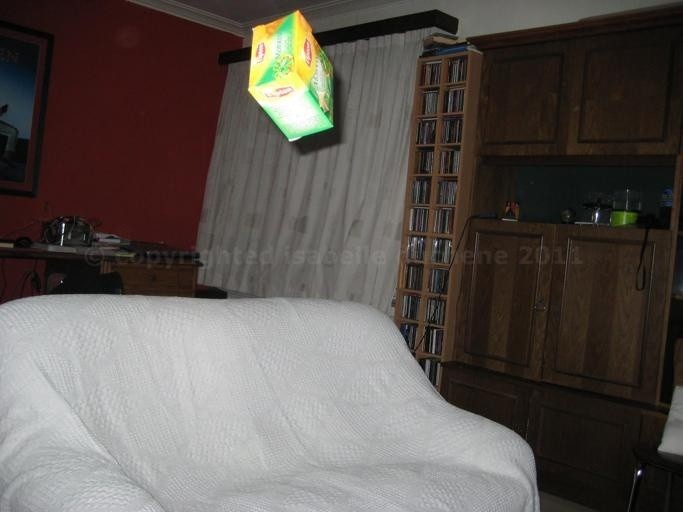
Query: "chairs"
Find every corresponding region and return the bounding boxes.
[50,271,123,295]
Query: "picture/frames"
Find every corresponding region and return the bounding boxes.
[0,20,55,200]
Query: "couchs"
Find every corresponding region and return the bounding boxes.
[0,296,543,512]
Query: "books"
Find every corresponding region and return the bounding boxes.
[90,232,133,250]
[396,30,478,398]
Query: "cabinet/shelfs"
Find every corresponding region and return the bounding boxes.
[443,366,642,512]
[454,215,672,404]
[480,0,682,165]
[393,48,482,395]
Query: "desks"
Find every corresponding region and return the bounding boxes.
[0,239,200,293]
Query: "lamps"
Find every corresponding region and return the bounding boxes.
[248,9,336,143]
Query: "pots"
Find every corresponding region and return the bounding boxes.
[581,203,640,227]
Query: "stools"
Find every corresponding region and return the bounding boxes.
[626,446,683,512]
